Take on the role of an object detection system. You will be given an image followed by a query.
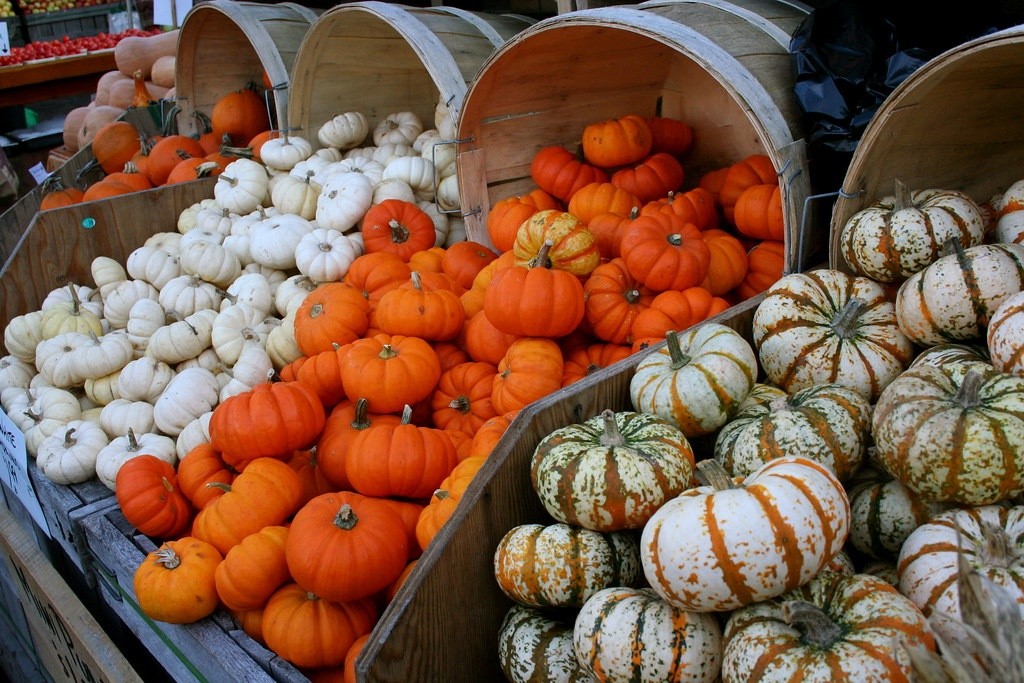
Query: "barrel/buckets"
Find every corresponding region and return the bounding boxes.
[428,1,814,282]
[283,0,538,158]
[827,22,1023,276]
[174,0,329,139]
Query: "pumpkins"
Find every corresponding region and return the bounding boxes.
[1,30,1024,682]
